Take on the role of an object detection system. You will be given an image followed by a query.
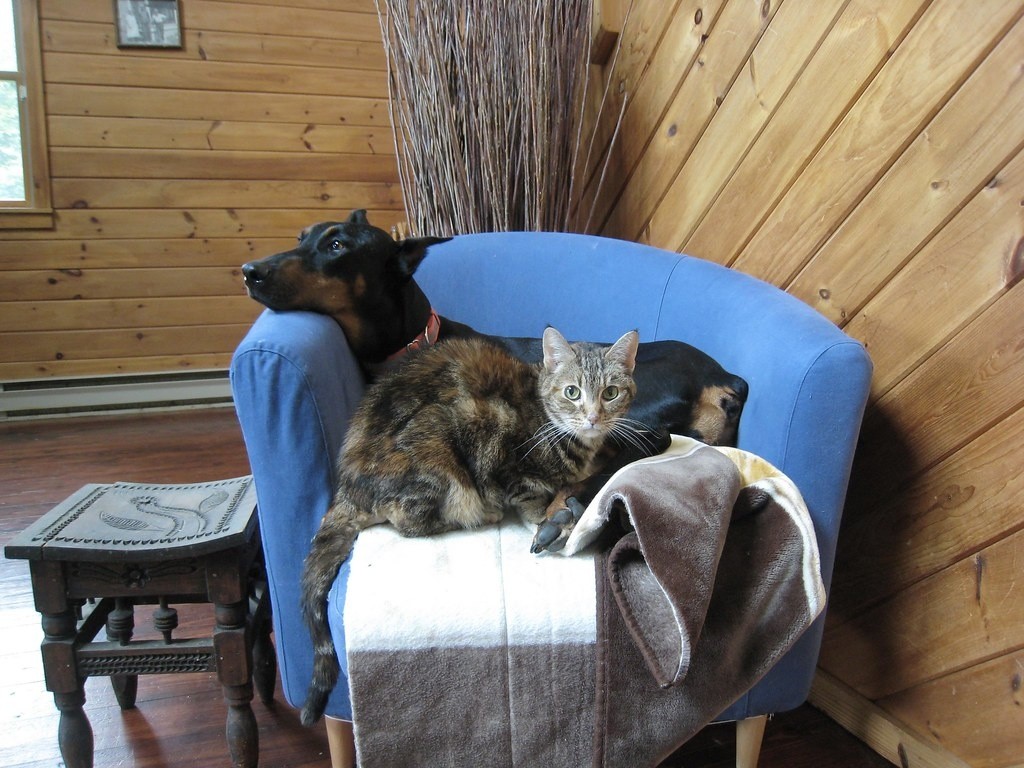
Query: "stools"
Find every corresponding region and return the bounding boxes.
[3,474,278,768]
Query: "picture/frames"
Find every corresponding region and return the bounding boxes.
[111,0,184,51]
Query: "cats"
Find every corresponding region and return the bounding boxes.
[296,326,641,728]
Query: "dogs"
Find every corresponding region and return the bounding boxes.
[241,210,749,447]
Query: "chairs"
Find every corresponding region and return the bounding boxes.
[228,231,876,768]
